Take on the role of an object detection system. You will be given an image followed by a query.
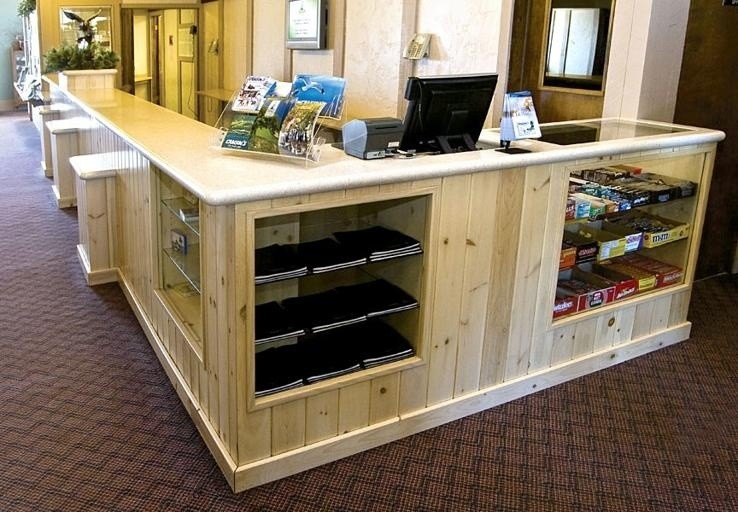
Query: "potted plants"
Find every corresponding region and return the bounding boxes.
[43,46,122,91]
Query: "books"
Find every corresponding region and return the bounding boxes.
[280,338,363,385]
[336,275,420,320]
[286,236,362,277]
[331,321,415,370]
[283,289,366,335]
[333,224,419,263]
[242,243,308,286]
[222,73,346,164]
[245,299,307,346]
[247,344,304,400]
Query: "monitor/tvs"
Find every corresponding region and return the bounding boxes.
[397,72,499,156]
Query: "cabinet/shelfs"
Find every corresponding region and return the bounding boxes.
[41,70,726,495]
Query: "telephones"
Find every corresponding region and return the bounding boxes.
[207,37,219,54]
[401,32,432,77]
